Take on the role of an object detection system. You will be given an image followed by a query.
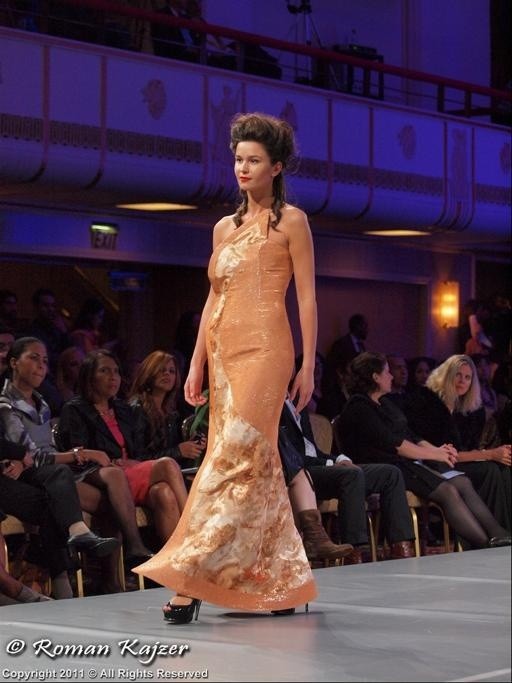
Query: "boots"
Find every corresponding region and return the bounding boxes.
[297,509,354,561]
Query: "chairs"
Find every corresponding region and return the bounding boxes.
[1,413,458,608]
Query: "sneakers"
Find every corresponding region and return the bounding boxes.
[345,542,417,562]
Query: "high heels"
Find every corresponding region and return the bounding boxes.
[271,598,309,614]
[63,529,119,559]
[162,591,204,624]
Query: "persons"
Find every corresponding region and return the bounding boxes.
[129,113,319,625]
